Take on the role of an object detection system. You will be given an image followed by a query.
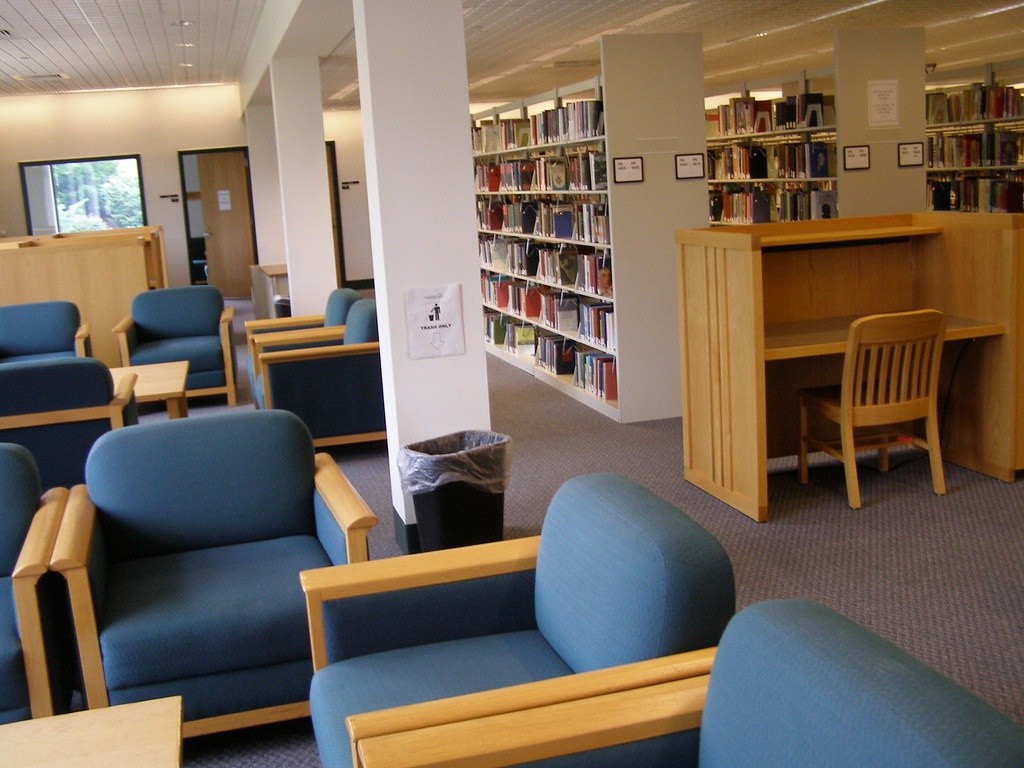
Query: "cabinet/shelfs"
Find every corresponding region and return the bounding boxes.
[927,115,1024,213]
[476,30,712,423]
[705,124,839,221]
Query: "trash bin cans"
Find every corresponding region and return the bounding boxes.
[402,428,510,553]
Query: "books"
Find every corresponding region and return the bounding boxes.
[706,93,838,224]
[925,81,1024,213]
[467,99,618,401]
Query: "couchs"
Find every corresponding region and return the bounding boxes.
[0,302,92,362]
[0,442,73,725]
[47,408,378,741]
[244,288,362,407]
[114,285,240,411]
[366,594,1024,766]
[299,471,737,768]
[255,299,387,448]
[1,353,140,491]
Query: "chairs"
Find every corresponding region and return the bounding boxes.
[799,309,948,509]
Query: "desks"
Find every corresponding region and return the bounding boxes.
[674,210,1024,525]
[110,360,188,422]
[260,265,287,317]
[0,695,184,768]
